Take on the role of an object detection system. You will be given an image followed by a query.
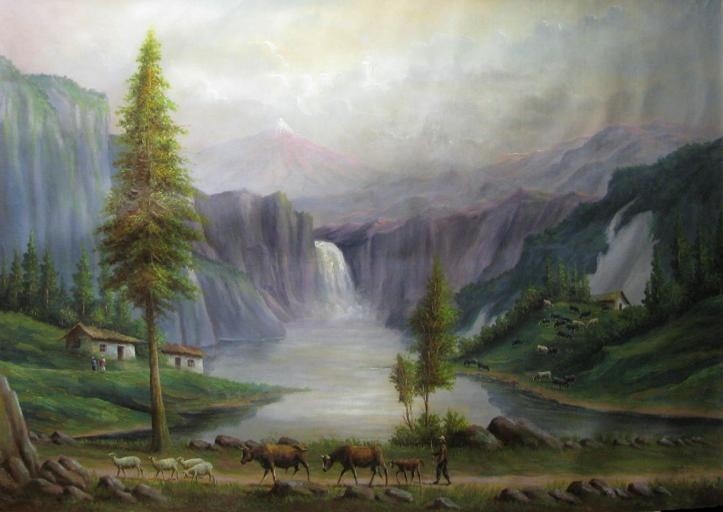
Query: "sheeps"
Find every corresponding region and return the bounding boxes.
[182,462,217,485]
[108,452,144,479]
[176,456,204,470]
[147,456,179,481]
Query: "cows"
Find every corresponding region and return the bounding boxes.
[512,339,522,345]
[320,443,389,488]
[241,442,310,487]
[536,343,558,356]
[529,370,576,393]
[387,458,425,486]
[537,298,609,332]
[277,437,309,474]
[464,359,489,371]
